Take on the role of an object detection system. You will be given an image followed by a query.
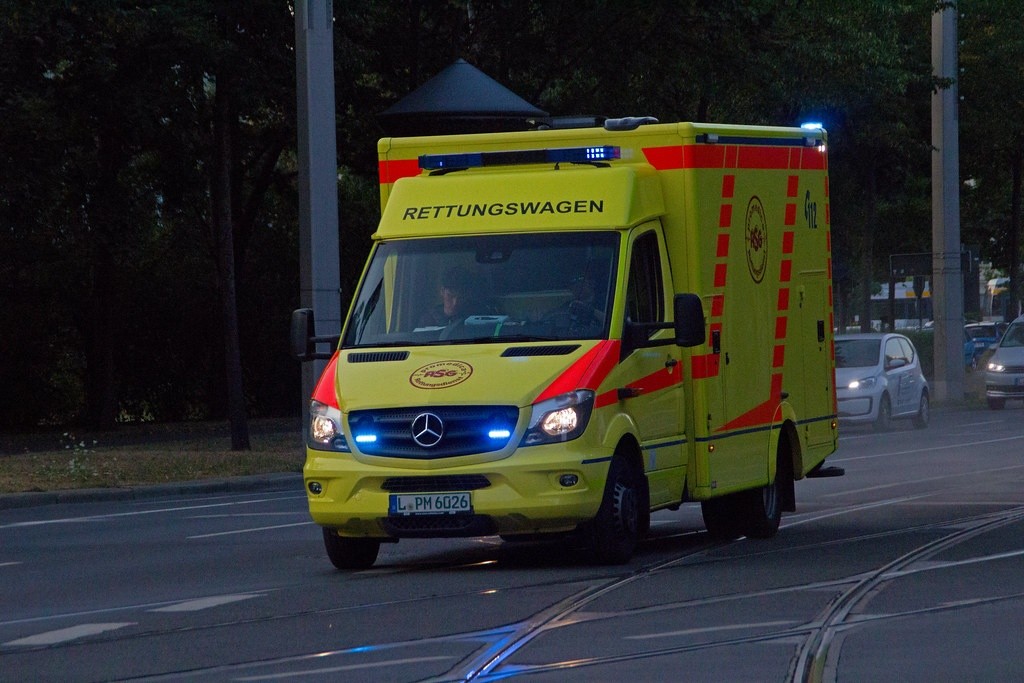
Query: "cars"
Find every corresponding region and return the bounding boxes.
[924,321,1008,371]
[833,333,931,432]
[984,314,1024,409]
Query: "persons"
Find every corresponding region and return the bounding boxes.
[418,269,479,327]
[560,259,607,326]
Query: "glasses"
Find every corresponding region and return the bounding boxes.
[568,275,585,285]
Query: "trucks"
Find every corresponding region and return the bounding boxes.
[291,117,846,571]
